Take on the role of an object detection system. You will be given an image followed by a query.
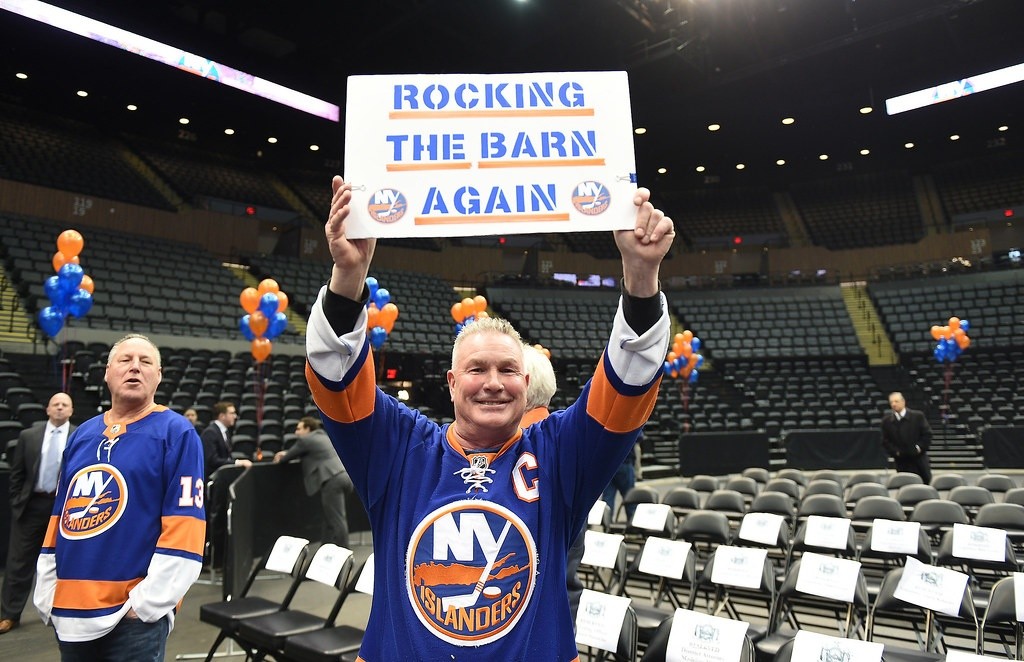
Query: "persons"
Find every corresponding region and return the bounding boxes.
[276,416,353,550]
[881,392,932,484]
[304,175,675,662]
[520,343,557,428]
[601,430,643,521]
[182,407,203,435]
[200,401,253,574]
[0,393,77,633]
[33,334,206,662]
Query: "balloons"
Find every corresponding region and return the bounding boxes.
[665,331,703,382]
[365,277,398,348]
[931,317,970,362]
[534,344,551,359]
[451,295,489,336]
[38,230,94,337]
[239,279,289,364]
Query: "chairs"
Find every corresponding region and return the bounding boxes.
[0,136,1024,662]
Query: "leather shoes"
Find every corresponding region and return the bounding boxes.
[0,619,20,633]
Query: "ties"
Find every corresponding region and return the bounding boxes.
[43,429,61,494]
[225,431,230,452]
